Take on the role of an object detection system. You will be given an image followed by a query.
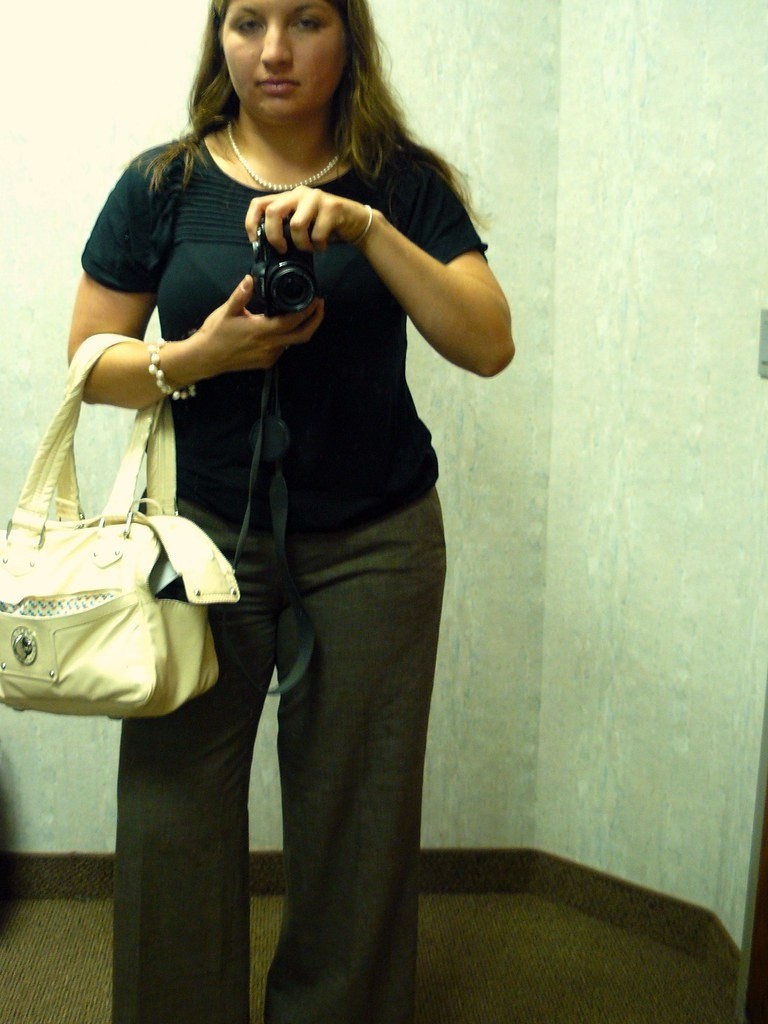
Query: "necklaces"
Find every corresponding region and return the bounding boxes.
[227,121,340,191]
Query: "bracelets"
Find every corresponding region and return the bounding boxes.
[148,337,196,399]
[347,205,373,247]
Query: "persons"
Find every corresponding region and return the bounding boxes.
[64,1,519,1024]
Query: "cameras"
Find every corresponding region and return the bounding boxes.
[250,217,316,320]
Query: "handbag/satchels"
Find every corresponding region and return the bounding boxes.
[0,335,241,723]
